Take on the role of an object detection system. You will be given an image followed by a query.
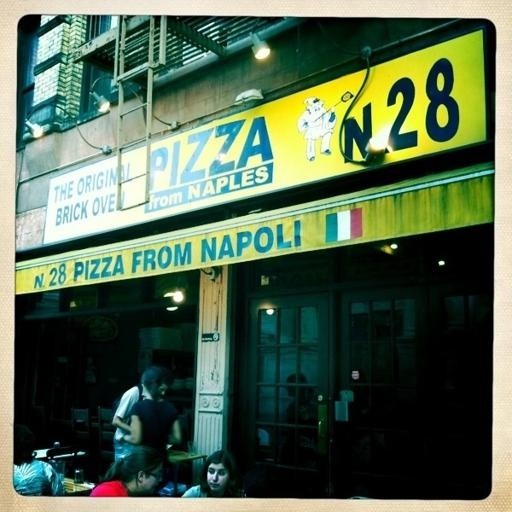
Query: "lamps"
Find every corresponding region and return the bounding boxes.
[243,31,272,61]
[338,48,395,167]
[23,74,180,156]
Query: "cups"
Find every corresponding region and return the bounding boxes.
[50,460,65,482]
[74,469,84,483]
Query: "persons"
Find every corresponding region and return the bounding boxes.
[13,364,243,497]
[285,372,315,457]
[330,428,378,499]
